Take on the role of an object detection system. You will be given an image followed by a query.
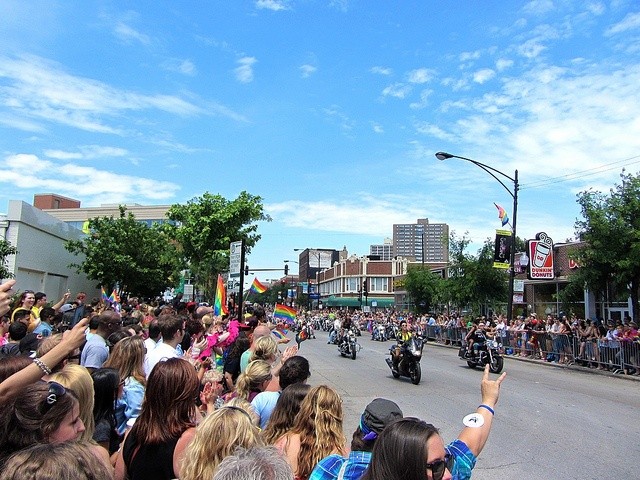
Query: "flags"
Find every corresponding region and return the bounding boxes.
[493,202,509,228]
[273,303,299,325]
[97,282,125,311]
[214,276,230,316]
[249,278,267,296]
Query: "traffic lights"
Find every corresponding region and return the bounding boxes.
[363,281,368,297]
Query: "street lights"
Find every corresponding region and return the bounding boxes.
[436,152,519,327]
[294,248,321,310]
[284,259,311,311]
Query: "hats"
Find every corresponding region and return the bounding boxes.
[58,304,84,313]
[530,313,538,319]
[186,302,198,309]
[202,313,213,324]
[20,333,44,352]
[196,306,214,316]
[242,317,258,331]
[360,398,403,435]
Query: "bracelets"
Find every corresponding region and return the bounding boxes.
[28,355,52,375]
[476,404,495,416]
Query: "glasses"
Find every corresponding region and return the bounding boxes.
[426,454,454,480]
[218,377,225,384]
[401,325,408,326]
[25,290,34,293]
[41,381,66,415]
[176,327,188,332]
[118,379,125,388]
[106,321,122,328]
[63,351,81,365]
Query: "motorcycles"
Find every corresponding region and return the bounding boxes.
[269,314,330,340]
[386,337,427,385]
[329,324,340,344]
[337,326,362,359]
[370,321,400,341]
[458,329,504,373]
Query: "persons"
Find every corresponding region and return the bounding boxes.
[251,355,311,434]
[0,280,16,317]
[186,407,259,480]
[112,357,208,479]
[247,317,290,346]
[185,381,224,427]
[0,356,32,381]
[2,380,86,456]
[50,365,135,479]
[10,290,40,333]
[182,346,212,386]
[72,285,106,336]
[254,308,279,330]
[459,310,640,376]
[361,305,459,368]
[176,320,205,361]
[107,285,158,335]
[0,322,28,359]
[38,293,71,320]
[142,323,163,362]
[0,318,91,417]
[32,307,55,342]
[38,334,95,449]
[80,311,124,374]
[9,309,31,343]
[243,307,254,322]
[102,335,149,452]
[207,445,295,480]
[224,337,249,394]
[234,303,238,316]
[240,325,298,374]
[264,384,350,480]
[158,292,216,325]
[250,337,281,393]
[1,437,116,479]
[228,302,232,314]
[360,417,455,480]
[19,333,43,358]
[225,360,271,416]
[30,292,46,318]
[106,332,131,360]
[308,362,506,479]
[214,320,231,371]
[0,315,12,346]
[290,306,361,360]
[221,314,230,332]
[263,384,311,445]
[142,315,208,383]
[89,368,125,457]
